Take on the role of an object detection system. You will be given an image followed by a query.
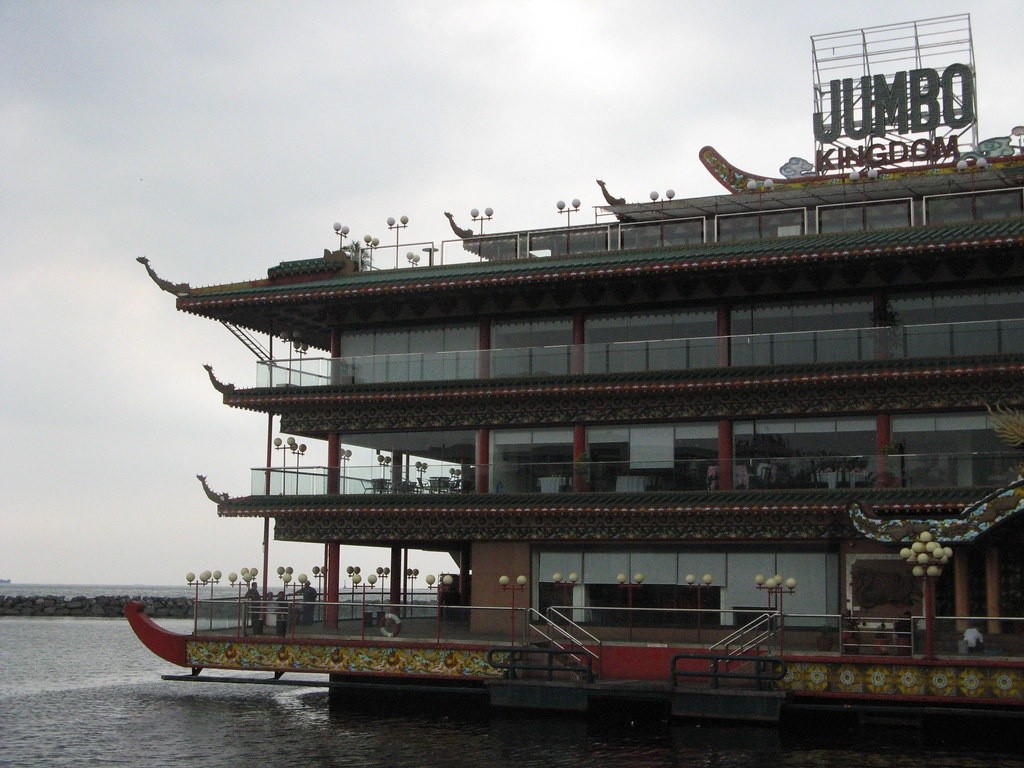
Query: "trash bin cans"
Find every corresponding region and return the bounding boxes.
[365,611,373,627]
[253,619,263,634]
[376,611,385,626]
[277,620,287,636]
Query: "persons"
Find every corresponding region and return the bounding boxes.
[295,581,317,625]
[963,623,984,653]
[245,582,266,629]
[894,610,917,637]
[262,591,289,613]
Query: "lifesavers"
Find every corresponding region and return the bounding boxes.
[378,614,402,637]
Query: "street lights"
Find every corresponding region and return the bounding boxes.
[275,436,351,495]
[277,567,293,596]
[353,574,378,639]
[313,566,328,620]
[406,568,419,618]
[616,573,644,642]
[346,565,360,617]
[333,157,989,269]
[279,331,309,386]
[686,574,713,642]
[499,576,527,646]
[553,573,578,638]
[378,455,462,488]
[228,568,259,636]
[186,571,221,636]
[900,532,952,658]
[282,573,307,638]
[427,575,453,642]
[377,567,390,610]
[754,575,797,655]
